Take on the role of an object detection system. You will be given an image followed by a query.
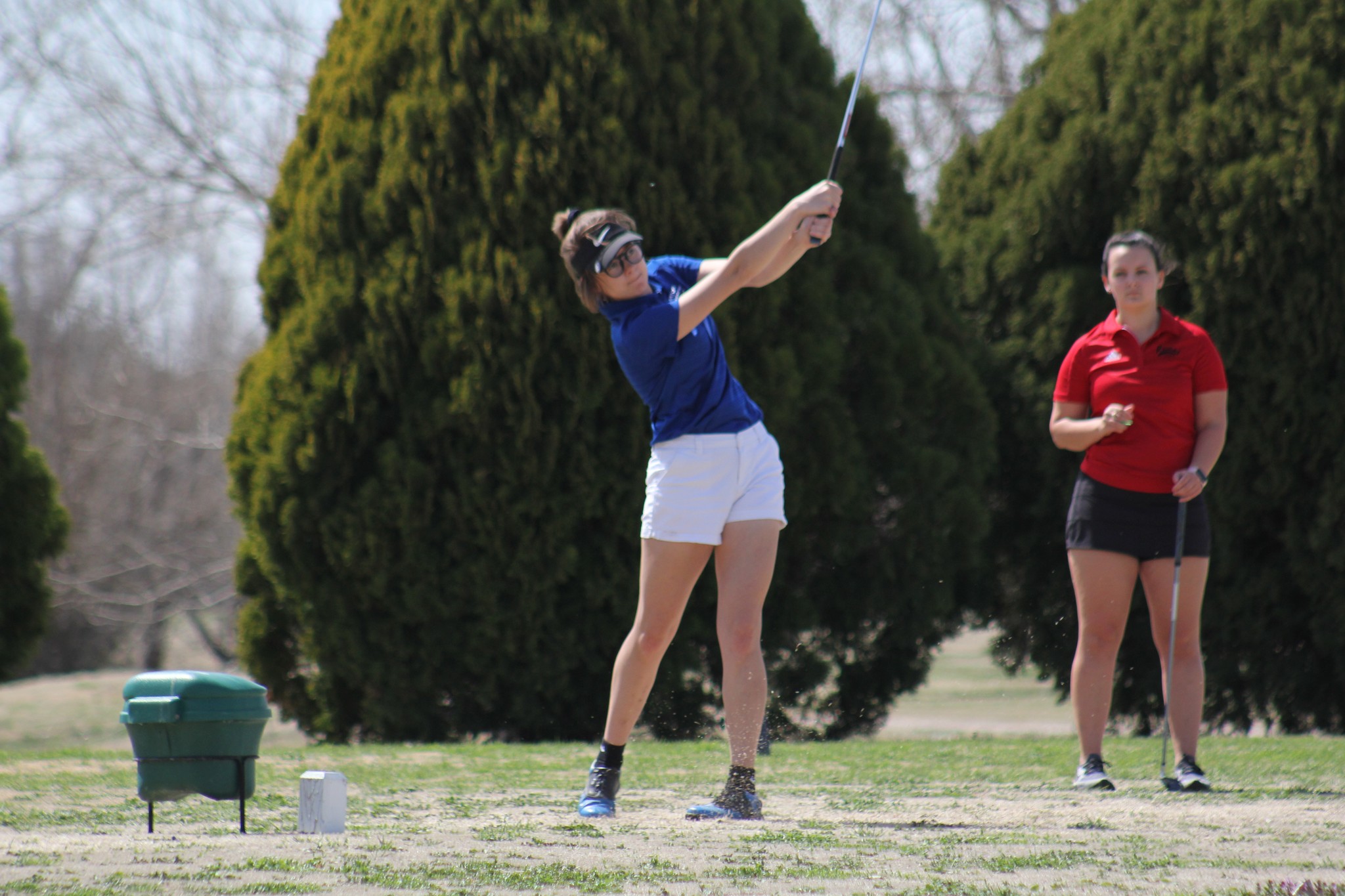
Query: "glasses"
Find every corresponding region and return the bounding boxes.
[601,241,643,278]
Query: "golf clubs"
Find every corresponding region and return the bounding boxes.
[1157,503,1190,793]
[806,1,888,247]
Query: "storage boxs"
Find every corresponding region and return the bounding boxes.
[300,772,346,832]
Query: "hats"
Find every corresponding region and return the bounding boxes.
[578,224,643,273]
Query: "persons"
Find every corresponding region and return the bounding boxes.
[552,181,843,819]
[1050,234,1228,795]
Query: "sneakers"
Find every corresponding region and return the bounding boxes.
[686,778,764,822]
[577,758,622,817]
[1073,753,1116,789]
[1170,750,1210,791]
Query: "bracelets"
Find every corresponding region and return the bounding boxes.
[1187,465,1208,486]
